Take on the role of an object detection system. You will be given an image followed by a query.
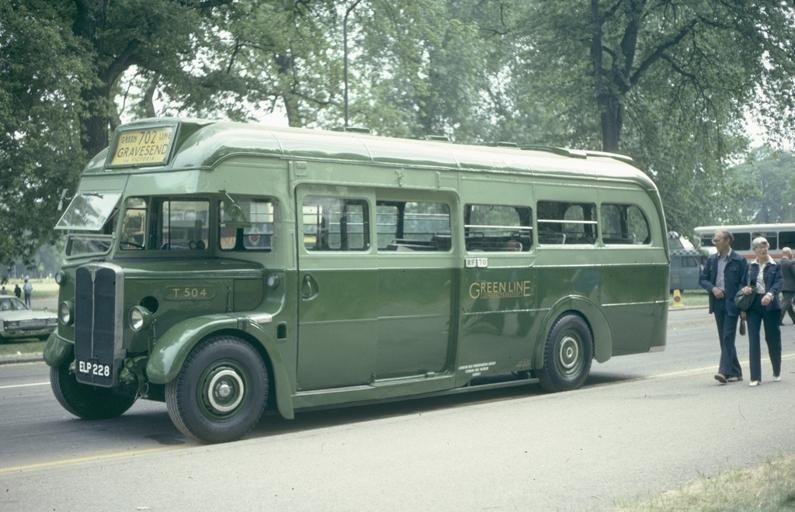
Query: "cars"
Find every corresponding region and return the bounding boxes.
[0,292,58,344]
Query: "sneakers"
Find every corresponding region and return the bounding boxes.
[727,375,743,382]
[714,372,728,383]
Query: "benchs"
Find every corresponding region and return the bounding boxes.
[384,228,638,253]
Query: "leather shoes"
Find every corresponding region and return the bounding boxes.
[770,372,781,382]
[749,379,761,386]
[779,317,795,326]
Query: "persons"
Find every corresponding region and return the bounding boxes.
[23,279,33,309]
[740,236,784,387]
[778,246,795,325]
[698,228,748,384]
[14,285,21,298]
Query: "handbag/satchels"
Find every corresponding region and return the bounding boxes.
[734,286,756,311]
[739,319,747,336]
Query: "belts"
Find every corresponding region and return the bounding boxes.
[755,293,766,297]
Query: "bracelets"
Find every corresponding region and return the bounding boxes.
[766,292,774,299]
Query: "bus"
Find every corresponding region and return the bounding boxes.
[35,113,671,446]
[693,222,795,265]
[667,231,709,294]
